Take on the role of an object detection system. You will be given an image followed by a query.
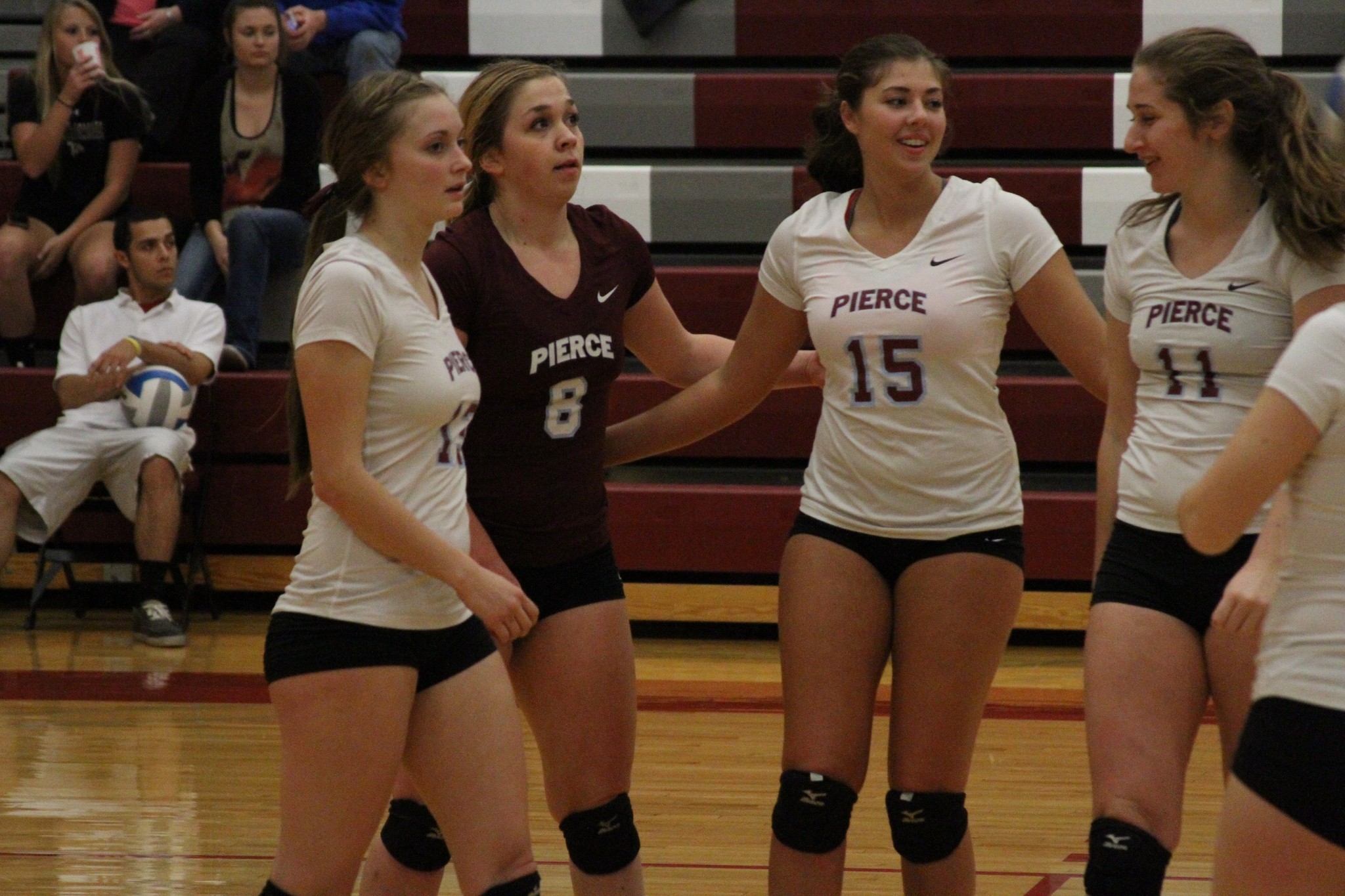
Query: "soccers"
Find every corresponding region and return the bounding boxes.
[120,365,191,429]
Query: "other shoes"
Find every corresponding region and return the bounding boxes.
[218,345,249,372]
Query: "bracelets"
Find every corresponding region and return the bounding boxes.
[165,8,173,22]
[122,337,141,356]
[57,97,75,108]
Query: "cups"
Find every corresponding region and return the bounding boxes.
[72,40,110,81]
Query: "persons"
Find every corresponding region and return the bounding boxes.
[1177,298,1345,896]
[1083,27,1345,896]
[358,60,826,896]
[0,0,407,370]
[0,205,228,647]
[256,68,540,896]
[605,34,1107,896]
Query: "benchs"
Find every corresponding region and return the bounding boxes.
[0,0,1345,577]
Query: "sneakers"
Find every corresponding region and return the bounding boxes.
[131,600,189,648]
